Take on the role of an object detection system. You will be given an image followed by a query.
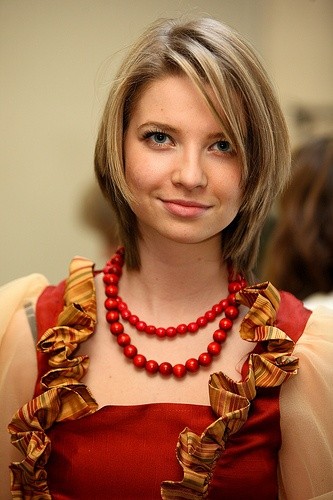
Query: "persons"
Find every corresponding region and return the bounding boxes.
[1,15,333,500]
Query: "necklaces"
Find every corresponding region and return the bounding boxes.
[101,246,248,380]
